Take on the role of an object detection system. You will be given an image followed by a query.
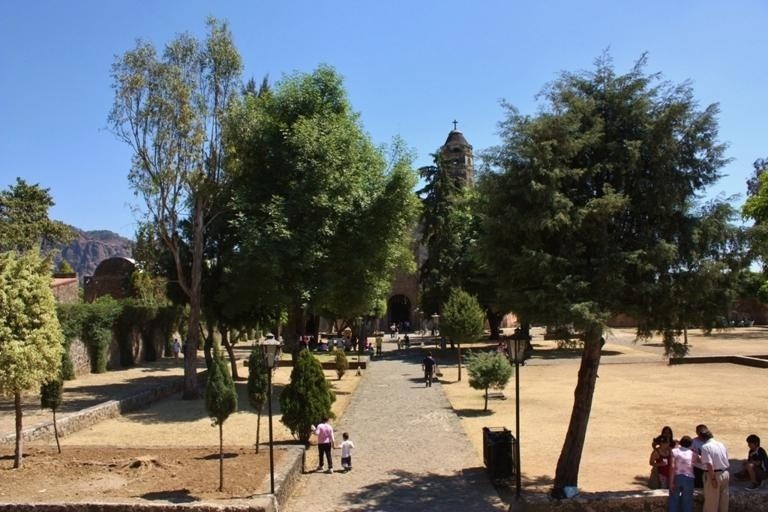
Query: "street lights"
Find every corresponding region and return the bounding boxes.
[262,331,282,496]
[416,304,440,371]
[507,327,530,500]
[355,316,364,377]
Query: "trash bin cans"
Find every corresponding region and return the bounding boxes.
[483,427,517,478]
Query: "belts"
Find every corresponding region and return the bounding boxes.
[704,469,723,472]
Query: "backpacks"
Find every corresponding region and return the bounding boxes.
[648,463,661,489]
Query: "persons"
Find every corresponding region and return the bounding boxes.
[172,339,182,365]
[376,334,382,356]
[494,326,512,359]
[311,413,335,473]
[668,435,702,511]
[439,336,445,349]
[698,428,731,512]
[733,433,768,491]
[648,436,671,489]
[332,431,354,470]
[181,339,189,362]
[299,332,375,359]
[652,425,680,450]
[689,424,711,489]
[526,342,533,359]
[388,318,413,352]
[422,350,437,388]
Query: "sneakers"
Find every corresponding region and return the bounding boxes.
[744,483,759,491]
[317,465,334,473]
